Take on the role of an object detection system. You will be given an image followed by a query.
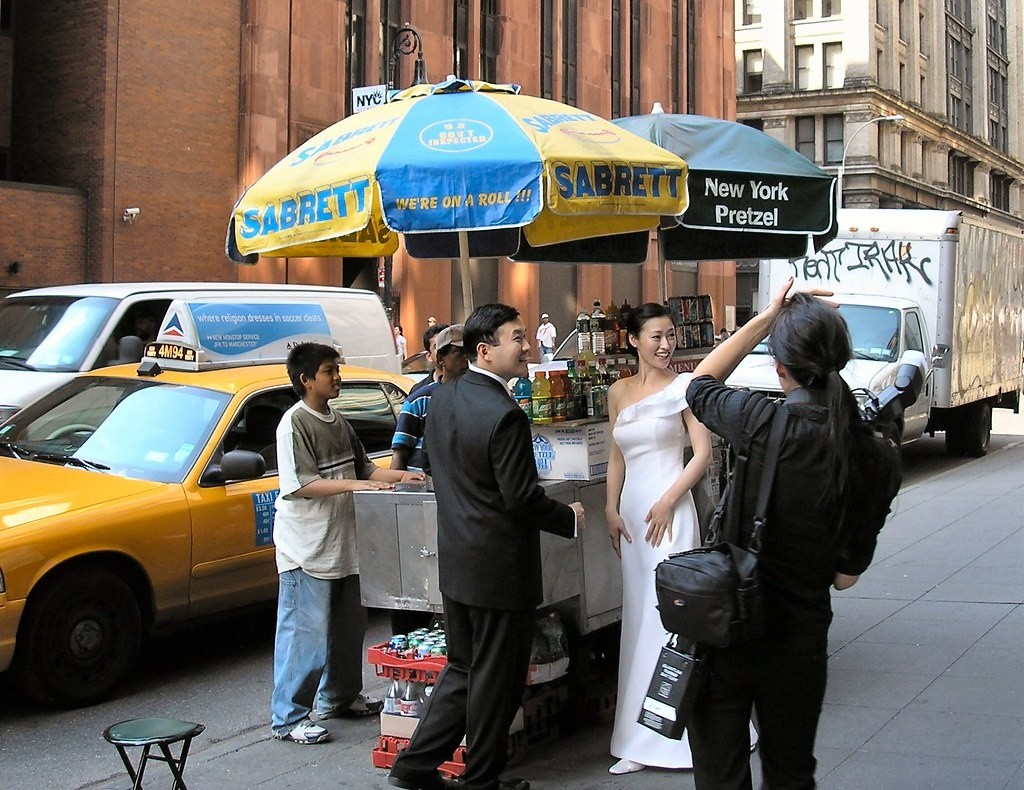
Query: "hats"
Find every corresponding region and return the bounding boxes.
[427,317,436,323]
[436,324,464,351]
[542,314,549,319]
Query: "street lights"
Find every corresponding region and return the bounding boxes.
[384,26,431,324]
[836,112,907,210]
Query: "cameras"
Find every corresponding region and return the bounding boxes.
[859,349,929,426]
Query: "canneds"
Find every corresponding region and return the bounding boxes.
[389,627,448,657]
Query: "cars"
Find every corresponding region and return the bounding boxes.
[0,339,419,706]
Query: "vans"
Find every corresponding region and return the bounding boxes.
[0,281,402,427]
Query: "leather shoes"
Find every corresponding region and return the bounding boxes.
[395,770,459,790]
[498,777,530,790]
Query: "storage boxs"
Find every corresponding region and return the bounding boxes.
[525,657,570,686]
[367,641,447,684]
[379,706,523,746]
[372,735,468,780]
[530,421,610,482]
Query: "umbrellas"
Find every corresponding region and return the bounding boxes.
[507,102,838,306]
[225,75,690,324]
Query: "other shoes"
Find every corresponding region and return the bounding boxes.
[609,758,646,775]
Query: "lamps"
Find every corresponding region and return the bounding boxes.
[123,208,140,224]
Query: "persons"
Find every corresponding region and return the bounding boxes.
[604,302,758,774]
[390,316,469,471]
[271,343,426,743]
[130,310,158,346]
[389,303,585,790]
[536,313,557,358]
[720,328,730,343]
[685,276,902,790]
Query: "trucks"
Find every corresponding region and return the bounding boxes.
[724,208,1023,457]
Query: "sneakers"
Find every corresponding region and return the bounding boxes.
[325,694,384,719]
[276,718,330,745]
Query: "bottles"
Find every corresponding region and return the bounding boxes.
[576,298,632,356]
[514,343,638,425]
[384,676,435,717]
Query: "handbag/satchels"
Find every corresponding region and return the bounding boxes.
[656,542,763,648]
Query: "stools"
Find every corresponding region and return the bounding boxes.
[103,717,206,790]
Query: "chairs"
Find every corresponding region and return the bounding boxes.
[223,404,286,471]
